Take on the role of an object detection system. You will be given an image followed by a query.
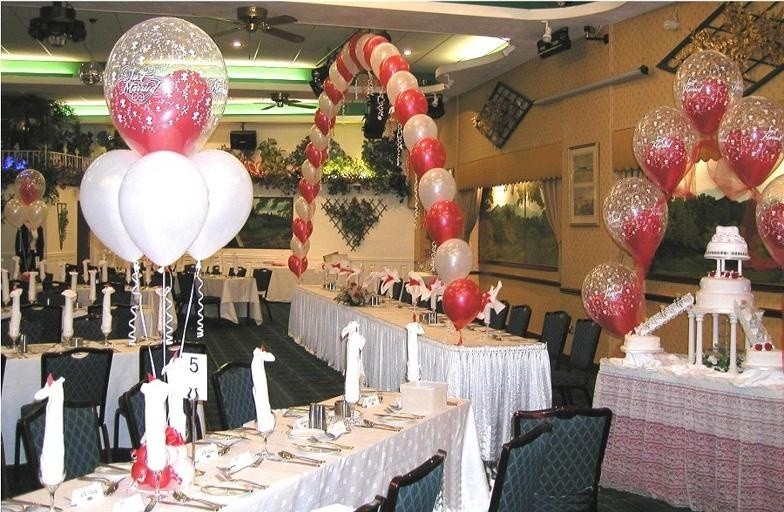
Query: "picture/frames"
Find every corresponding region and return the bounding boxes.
[565,140,601,228]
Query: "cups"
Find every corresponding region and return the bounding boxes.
[334,400,351,417]
[309,402,327,429]
[167,413,190,441]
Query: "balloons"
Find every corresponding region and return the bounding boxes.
[79,15,253,269]
[2,168,51,230]
[287,35,482,331]
[581,51,783,339]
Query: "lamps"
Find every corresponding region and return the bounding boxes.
[25,2,89,49]
[529,60,652,109]
[441,72,457,91]
[431,92,439,108]
[539,19,554,46]
[494,36,517,58]
[663,7,681,32]
[75,53,107,90]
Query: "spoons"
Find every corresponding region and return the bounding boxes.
[362,417,400,429]
[77,475,126,496]
[172,490,224,508]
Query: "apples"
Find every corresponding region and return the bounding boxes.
[133,428,184,488]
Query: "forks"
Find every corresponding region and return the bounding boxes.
[143,497,156,511]
[215,455,267,490]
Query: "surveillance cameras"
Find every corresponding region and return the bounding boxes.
[583,25,595,33]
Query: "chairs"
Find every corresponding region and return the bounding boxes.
[116,376,193,451]
[1,249,273,344]
[140,341,206,381]
[507,304,532,338]
[15,400,103,481]
[489,420,551,511]
[213,358,263,428]
[540,311,572,361]
[377,275,510,331]
[390,448,446,512]
[16,346,114,468]
[515,406,614,495]
[356,496,393,512]
[239,251,362,303]
[551,318,602,404]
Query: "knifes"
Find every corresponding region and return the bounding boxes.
[353,424,399,432]
[267,432,351,468]
[95,460,130,472]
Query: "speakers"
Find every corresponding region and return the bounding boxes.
[230,130,257,150]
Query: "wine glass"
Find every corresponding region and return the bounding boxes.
[143,446,169,499]
[11,322,113,357]
[253,410,276,457]
[37,455,68,511]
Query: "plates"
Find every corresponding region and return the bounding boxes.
[202,429,247,442]
[286,428,325,439]
[328,408,363,419]
[198,480,252,497]
[94,462,136,475]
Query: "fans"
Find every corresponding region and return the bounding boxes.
[249,91,316,112]
[190,5,307,45]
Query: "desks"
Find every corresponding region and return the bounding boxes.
[2,387,475,512]
[599,341,783,509]
[291,280,554,462]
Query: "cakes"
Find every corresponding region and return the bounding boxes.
[747,343,783,368]
[622,328,661,349]
[696,269,754,308]
[703,225,750,258]
